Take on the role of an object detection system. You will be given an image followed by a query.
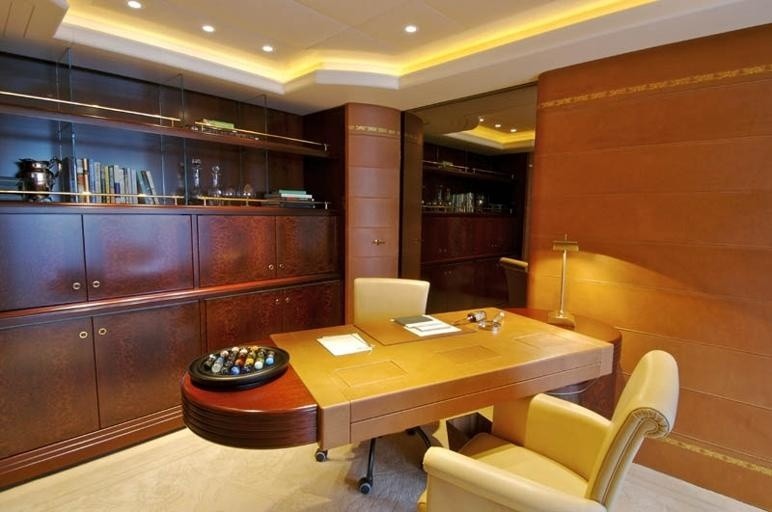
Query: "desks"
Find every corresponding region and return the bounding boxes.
[180,306,622,452]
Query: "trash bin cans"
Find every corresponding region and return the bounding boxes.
[446,412,492,452]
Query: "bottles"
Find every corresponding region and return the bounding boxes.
[437,184,452,214]
[22,157,62,205]
[191,157,256,209]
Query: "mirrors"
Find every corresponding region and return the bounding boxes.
[399,79,540,315]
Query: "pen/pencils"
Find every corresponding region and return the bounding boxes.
[203,346,276,374]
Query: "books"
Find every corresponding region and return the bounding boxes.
[260,187,316,209]
[61,156,161,205]
[394,314,461,338]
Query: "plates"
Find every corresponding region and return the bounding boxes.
[187,345,288,389]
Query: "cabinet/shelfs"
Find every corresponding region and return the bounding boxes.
[1,47,345,493]
[422,140,519,314]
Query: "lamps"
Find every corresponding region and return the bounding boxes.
[547,233,580,327]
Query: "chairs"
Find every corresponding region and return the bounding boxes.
[500,257,528,306]
[417,350,680,512]
[315,277,431,494]
